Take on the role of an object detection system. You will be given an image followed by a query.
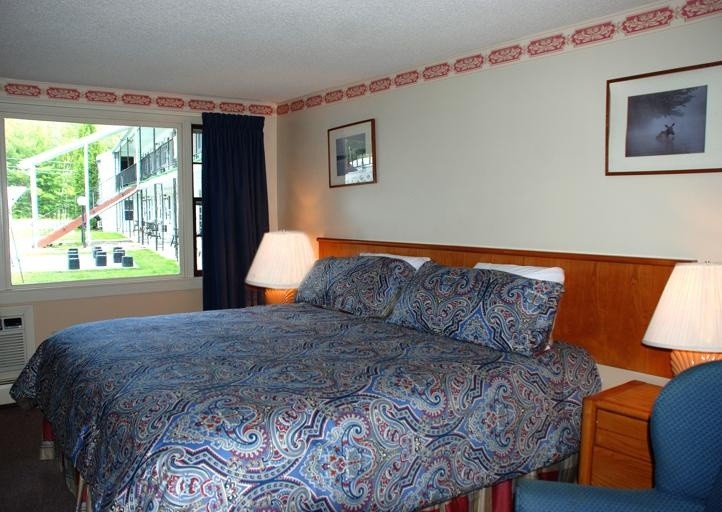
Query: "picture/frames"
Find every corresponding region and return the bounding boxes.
[328,118,378,189]
[605,60,722,176]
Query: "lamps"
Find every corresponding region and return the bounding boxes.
[640,260,722,376]
[245,229,316,304]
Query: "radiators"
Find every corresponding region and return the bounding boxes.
[0,304,36,405]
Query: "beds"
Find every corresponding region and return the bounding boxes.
[44,302,603,512]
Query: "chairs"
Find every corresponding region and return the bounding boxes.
[516,359,722,512]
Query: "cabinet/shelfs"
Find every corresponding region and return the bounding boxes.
[579,380,664,490]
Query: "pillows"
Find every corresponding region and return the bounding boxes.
[360,252,432,270]
[474,261,566,284]
[388,260,567,357]
[296,256,418,317]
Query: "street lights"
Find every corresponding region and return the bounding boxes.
[77,196,88,247]
